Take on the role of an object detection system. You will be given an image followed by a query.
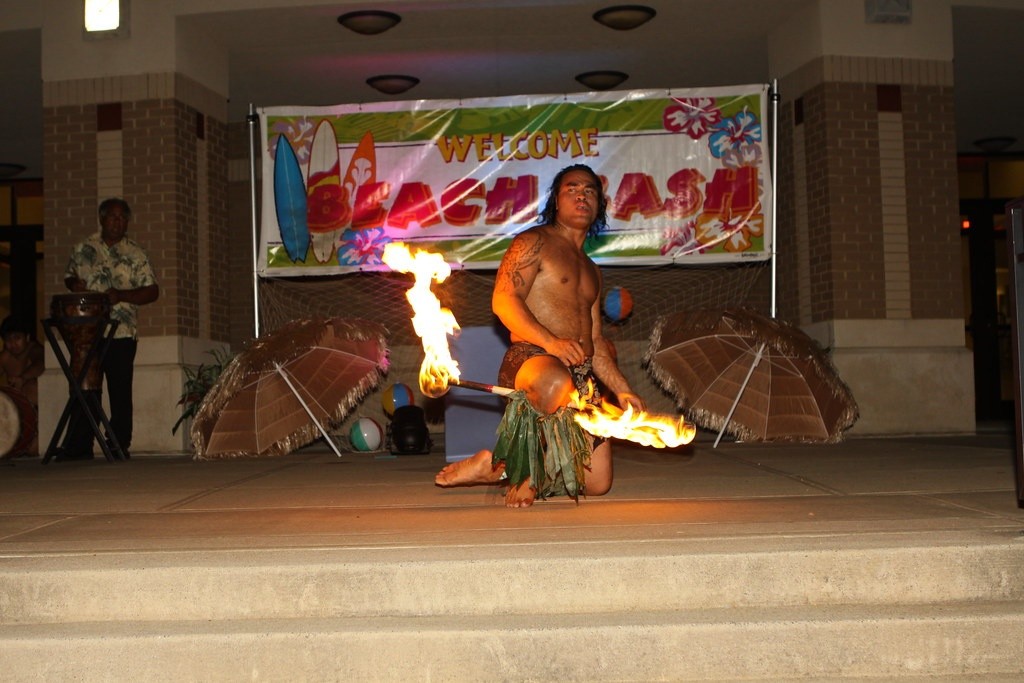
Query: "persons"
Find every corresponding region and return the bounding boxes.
[436,163,647,507]
[0,312,44,456]
[62,197,160,462]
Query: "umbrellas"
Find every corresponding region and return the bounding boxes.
[644,305,861,449]
[189,312,391,462]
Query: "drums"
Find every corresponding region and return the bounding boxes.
[0,384,36,460]
[49,290,111,393]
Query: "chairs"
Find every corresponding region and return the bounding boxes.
[445,324,515,463]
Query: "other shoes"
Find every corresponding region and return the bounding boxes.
[111,445,130,460]
[54,447,94,460]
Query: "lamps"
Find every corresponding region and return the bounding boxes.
[591,5,657,31]
[336,9,402,36]
[574,71,630,90]
[365,74,420,95]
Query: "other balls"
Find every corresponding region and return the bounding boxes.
[602,286,634,322]
[382,383,415,417]
[350,417,384,452]
